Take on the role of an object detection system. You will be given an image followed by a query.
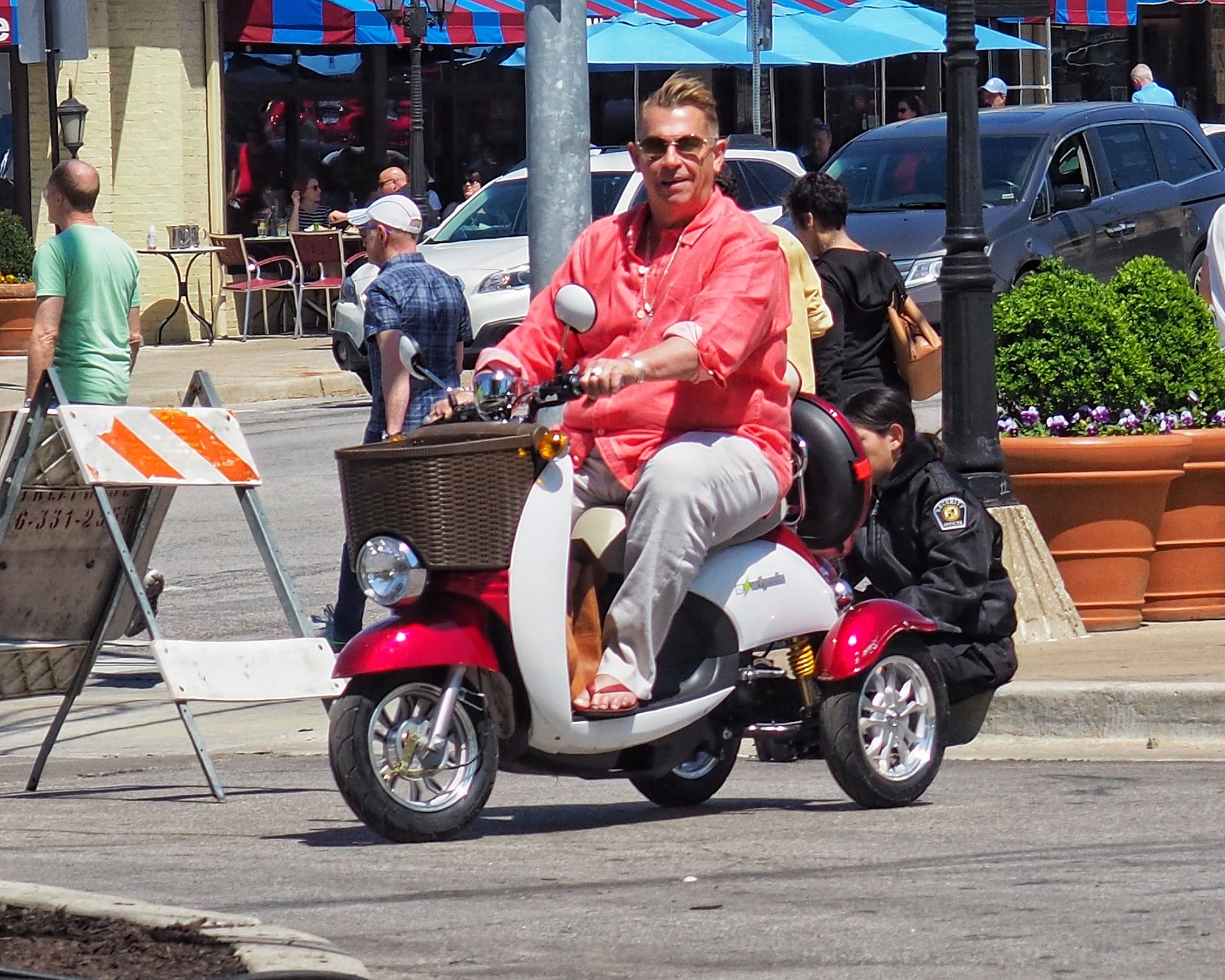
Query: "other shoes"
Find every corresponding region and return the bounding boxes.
[311,604,354,653]
[124,567,165,638]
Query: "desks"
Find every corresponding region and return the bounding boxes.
[137,247,227,346]
[242,233,363,333]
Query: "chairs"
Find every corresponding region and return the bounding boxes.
[203,232,303,342]
[287,229,346,339]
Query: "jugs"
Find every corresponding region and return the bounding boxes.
[190,224,206,248]
[166,225,193,249]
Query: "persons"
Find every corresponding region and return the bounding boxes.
[1130,64,1176,106]
[285,167,511,301]
[714,77,1019,762]
[308,195,476,654]
[1200,204,1225,348]
[26,160,165,638]
[426,69,793,718]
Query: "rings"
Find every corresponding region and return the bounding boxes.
[591,367,602,375]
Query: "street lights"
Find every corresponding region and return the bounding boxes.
[371,0,460,245]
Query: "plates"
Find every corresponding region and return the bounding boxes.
[344,230,359,235]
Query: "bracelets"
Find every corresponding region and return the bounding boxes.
[455,386,475,392]
[621,352,645,384]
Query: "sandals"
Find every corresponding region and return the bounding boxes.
[573,680,639,717]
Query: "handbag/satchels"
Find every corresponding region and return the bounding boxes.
[886,276,944,401]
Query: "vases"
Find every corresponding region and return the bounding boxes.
[0,285,39,355]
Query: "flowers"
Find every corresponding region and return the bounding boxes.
[0,208,34,284]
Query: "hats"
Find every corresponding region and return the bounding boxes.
[980,77,1007,95]
[347,195,423,234]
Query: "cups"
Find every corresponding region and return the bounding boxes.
[256,218,268,238]
[276,218,287,237]
[314,222,320,232]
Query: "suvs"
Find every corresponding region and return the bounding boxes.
[330,131,808,397]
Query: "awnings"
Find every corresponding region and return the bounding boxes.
[239,0,1137,44]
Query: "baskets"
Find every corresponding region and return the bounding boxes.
[334,420,548,575]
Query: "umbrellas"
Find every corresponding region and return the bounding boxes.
[500,0,1048,149]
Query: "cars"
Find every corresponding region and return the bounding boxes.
[764,100,1225,355]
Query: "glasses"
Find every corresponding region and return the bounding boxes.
[378,179,396,187]
[898,108,912,113]
[636,134,718,161]
[463,178,481,185]
[813,125,830,132]
[306,184,321,190]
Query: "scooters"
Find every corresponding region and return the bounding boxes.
[324,283,962,844]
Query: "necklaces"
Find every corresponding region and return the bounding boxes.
[642,224,682,313]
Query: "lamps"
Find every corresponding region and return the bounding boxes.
[57,79,89,160]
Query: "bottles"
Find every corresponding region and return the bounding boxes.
[147,225,156,249]
